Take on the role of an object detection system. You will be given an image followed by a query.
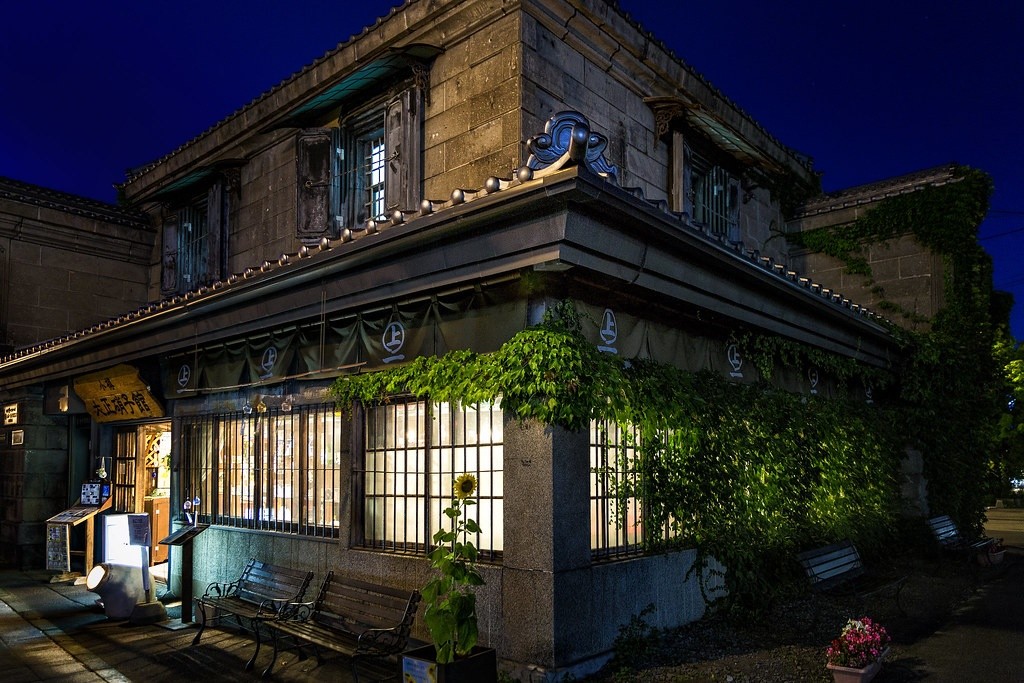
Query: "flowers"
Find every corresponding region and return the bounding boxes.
[420,471,484,662]
[987,538,1004,553]
[827,617,891,668]
[96,464,107,479]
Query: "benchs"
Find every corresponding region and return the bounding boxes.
[262,570,422,683]
[922,510,1004,579]
[192,558,314,673]
[785,538,914,630]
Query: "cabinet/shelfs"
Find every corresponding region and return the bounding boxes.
[144,497,170,566]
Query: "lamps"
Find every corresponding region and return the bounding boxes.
[59,384,68,412]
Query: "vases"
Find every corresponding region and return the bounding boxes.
[827,647,890,683]
[980,549,1006,564]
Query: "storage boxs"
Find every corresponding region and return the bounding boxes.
[396,641,497,683]
